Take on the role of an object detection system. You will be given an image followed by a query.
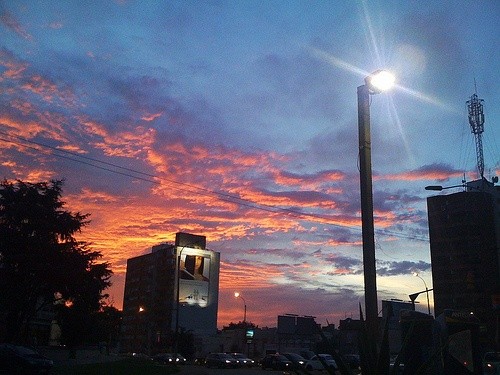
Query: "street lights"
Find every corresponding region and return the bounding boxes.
[349,69,396,375]
[410,271,431,315]
[235,292,248,326]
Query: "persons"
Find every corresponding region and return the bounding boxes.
[181,254,208,281]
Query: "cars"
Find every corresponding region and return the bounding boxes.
[260,352,318,372]
[305,355,338,373]
[345,355,362,371]
[390,355,405,368]
[206,353,262,369]
[104,352,207,366]
